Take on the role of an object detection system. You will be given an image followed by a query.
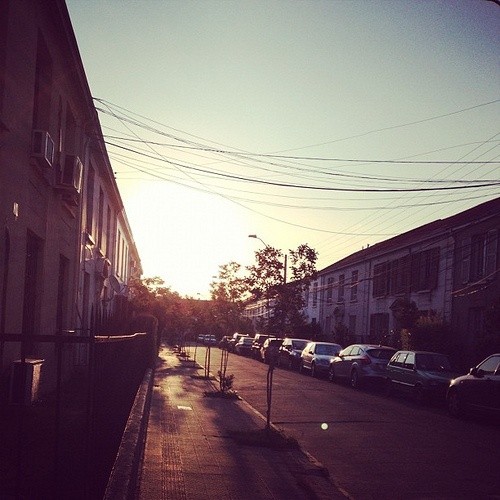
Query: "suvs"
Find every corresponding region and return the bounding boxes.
[227,332,250,353]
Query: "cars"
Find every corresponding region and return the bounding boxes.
[235,337,254,356]
[384,350,462,404]
[261,338,284,364]
[204,334,217,347]
[300,342,343,377]
[328,344,398,388]
[445,354,500,428]
[277,339,312,370]
[196,334,205,342]
[219,336,232,351]
[251,334,275,360]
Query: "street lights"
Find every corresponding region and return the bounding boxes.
[248,234,287,285]
